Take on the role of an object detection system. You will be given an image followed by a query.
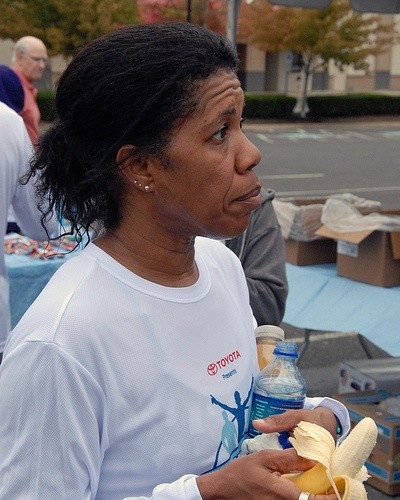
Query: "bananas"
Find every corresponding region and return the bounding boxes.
[280,416,378,499]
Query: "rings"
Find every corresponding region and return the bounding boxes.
[298,491,312,500]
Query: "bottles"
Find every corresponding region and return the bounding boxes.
[242,341,307,455]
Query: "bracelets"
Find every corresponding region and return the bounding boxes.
[335,415,344,436]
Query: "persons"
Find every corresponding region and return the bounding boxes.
[11,36,49,150]
[218,186,288,326]
[0,19,338,500]
[0,100,61,365]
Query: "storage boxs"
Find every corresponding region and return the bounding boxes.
[270,193,400,289]
[330,390,400,496]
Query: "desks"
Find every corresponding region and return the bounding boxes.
[281,261,400,368]
[4,230,94,331]
